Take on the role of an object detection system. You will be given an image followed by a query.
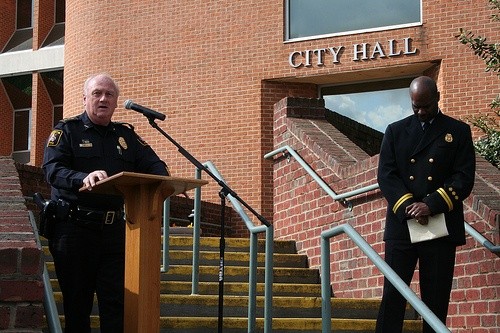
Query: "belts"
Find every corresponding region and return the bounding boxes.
[56,203,123,226]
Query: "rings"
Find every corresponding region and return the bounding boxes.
[412,212,415,217]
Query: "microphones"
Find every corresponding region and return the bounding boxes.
[124,100,166,121]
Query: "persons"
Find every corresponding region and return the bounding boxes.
[373,76,477,333]
[30,70,170,333]
